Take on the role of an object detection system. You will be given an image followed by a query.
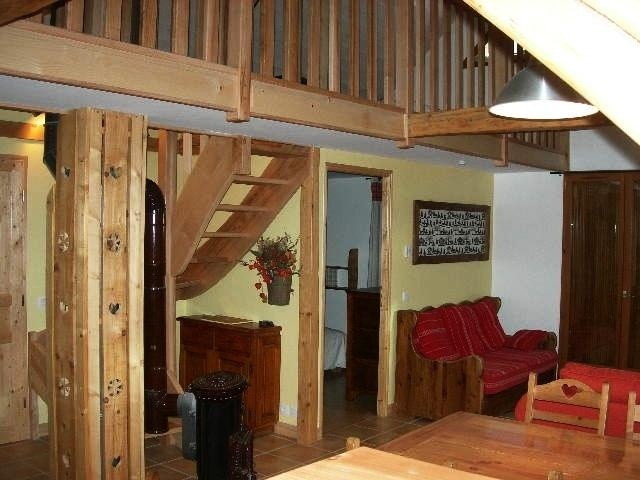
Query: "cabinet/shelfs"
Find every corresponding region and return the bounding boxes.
[558,170,640,379]
[176,313,282,439]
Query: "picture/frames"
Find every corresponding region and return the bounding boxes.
[412,200,491,265]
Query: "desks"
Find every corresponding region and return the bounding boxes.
[373,409,640,480]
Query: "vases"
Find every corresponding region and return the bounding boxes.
[268,274,293,306]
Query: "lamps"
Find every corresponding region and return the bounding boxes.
[488,61,600,120]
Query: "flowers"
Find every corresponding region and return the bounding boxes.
[234,232,303,302]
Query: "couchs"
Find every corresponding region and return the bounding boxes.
[394,296,559,422]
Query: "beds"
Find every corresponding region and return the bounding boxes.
[325,248,359,378]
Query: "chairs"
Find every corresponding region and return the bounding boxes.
[526,371,640,443]
